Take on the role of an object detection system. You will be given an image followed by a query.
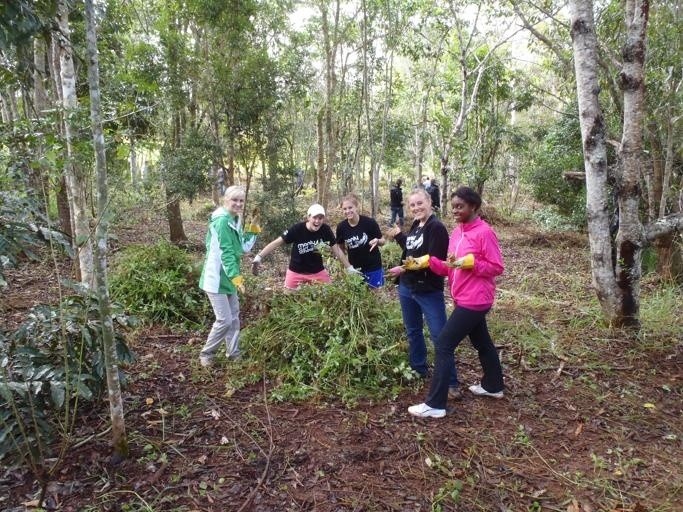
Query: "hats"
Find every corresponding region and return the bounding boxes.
[308,204,325,217]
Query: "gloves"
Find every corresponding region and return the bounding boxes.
[402,254,430,269]
[231,276,246,294]
[249,207,262,233]
[251,255,261,276]
[345,265,360,276]
[443,255,475,269]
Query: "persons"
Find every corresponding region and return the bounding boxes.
[334,196,386,291]
[401,186,506,419]
[194,184,262,370]
[387,179,404,227]
[385,187,462,401]
[425,179,440,212]
[421,177,431,190]
[252,203,362,292]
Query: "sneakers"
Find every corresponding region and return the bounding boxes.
[200,357,212,367]
[408,403,446,418]
[469,385,504,400]
[448,388,460,401]
